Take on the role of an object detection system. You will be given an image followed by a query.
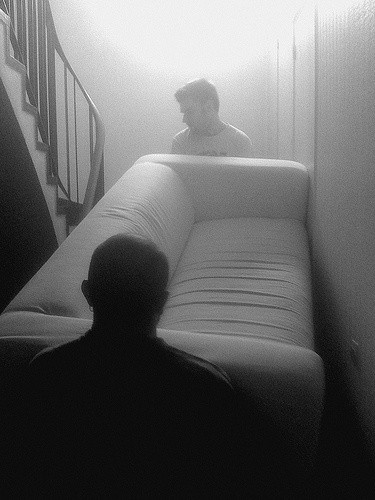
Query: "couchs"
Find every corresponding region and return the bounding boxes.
[0,155,323,482]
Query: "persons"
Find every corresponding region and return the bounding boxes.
[171,78,252,158]
[2,234,262,500]
